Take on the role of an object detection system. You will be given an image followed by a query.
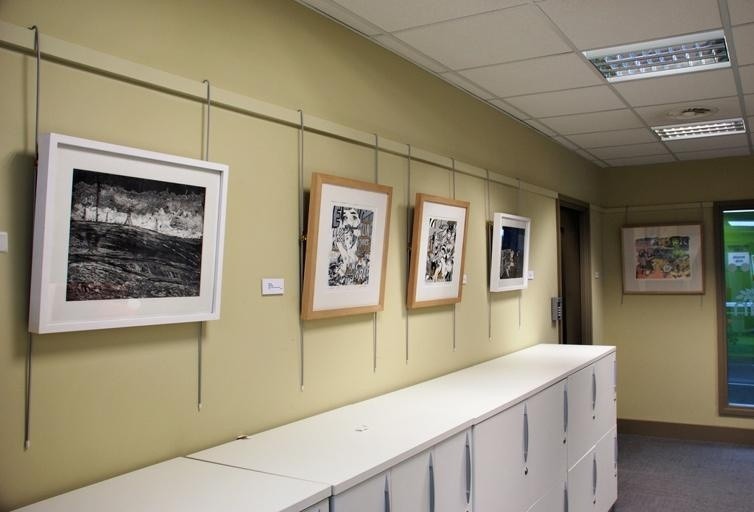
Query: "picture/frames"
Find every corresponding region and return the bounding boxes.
[617,222,705,296]
[487,211,531,293]
[299,170,393,323]
[26,130,229,336]
[404,190,470,311]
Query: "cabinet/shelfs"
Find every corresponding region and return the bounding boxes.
[566,350,618,512]
[330,420,472,511]
[472,376,568,510]
